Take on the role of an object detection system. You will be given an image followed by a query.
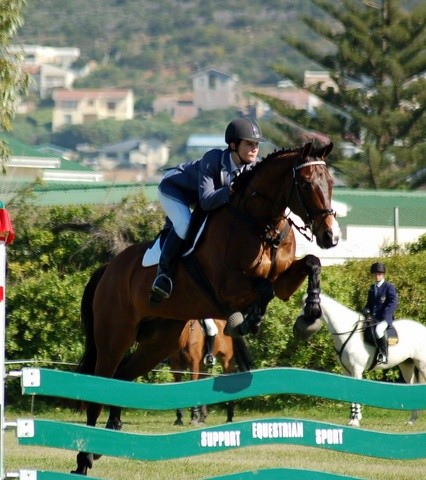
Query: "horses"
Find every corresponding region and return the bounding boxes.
[71,134,426,477]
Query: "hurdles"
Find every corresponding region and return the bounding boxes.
[4,367,426,479]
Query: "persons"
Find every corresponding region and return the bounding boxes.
[198,318,219,367]
[148,118,268,308]
[361,262,397,365]
